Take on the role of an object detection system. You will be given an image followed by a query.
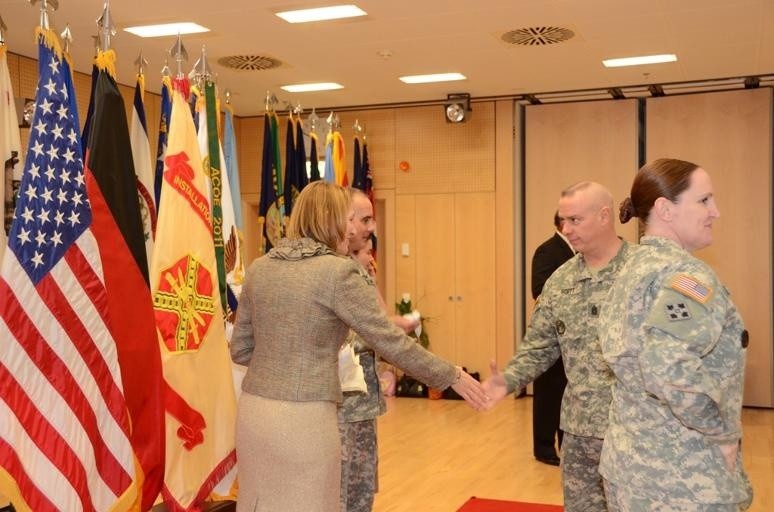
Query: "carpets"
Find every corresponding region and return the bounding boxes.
[453,496,564,512]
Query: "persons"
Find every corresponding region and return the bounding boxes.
[350,228,379,276]
[226,179,489,511]
[528,206,568,468]
[485,180,635,511]
[334,188,419,511]
[596,157,757,511]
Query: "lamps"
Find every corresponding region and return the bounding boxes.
[443,91,472,125]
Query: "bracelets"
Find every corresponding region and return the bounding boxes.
[452,365,462,383]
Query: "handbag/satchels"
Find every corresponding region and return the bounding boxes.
[393,366,485,403]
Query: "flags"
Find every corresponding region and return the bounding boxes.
[77,6,165,511]
[146,31,240,511]
[189,81,247,396]
[151,61,175,203]
[124,42,157,234]
[0,0,144,511]
[258,112,377,275]
[0,17,23,268]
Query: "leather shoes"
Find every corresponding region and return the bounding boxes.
[534,452,561,466]
